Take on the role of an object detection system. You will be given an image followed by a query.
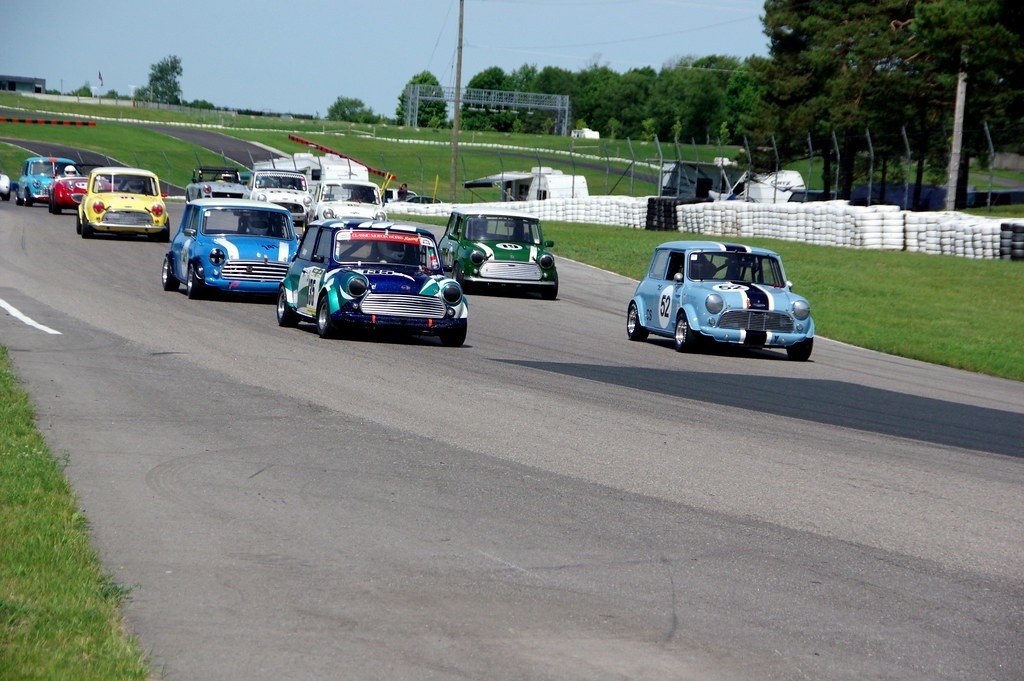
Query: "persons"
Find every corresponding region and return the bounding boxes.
[246,214,269,236]
[376,240,407,264]
[63,165,77,177]
[127,180,145,194]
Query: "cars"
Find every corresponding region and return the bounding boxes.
[185,165,245,203]
[48,162,118,215]
[242,169,313,226]
[625,240,816,362]
[404,195,444,206]
[438,210,558,300]
[76,166,172,244]
[385,188,418,204]
[301,178,387,234]
[274,217,468,347]
[160,198,303,302]
[14,157,82,207]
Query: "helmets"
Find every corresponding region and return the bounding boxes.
[281,178,294,188]
[127,180,144,193]
[691,256,707,278]
[378,240,407,261]
[247,215,269,234]
[64,165,77,177]
